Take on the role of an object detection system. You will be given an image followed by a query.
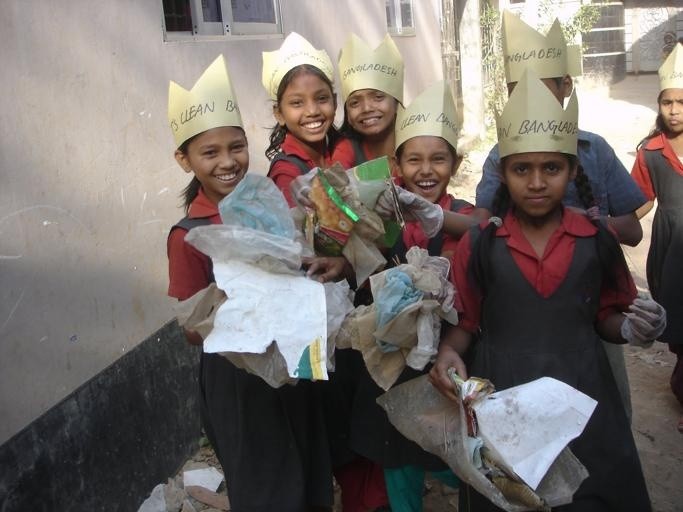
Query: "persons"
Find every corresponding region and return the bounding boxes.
[255,31,473,512]
[628,40,683,434]
[427,67,667,512]
[162,52,335,512]
[474,4,647,431]
